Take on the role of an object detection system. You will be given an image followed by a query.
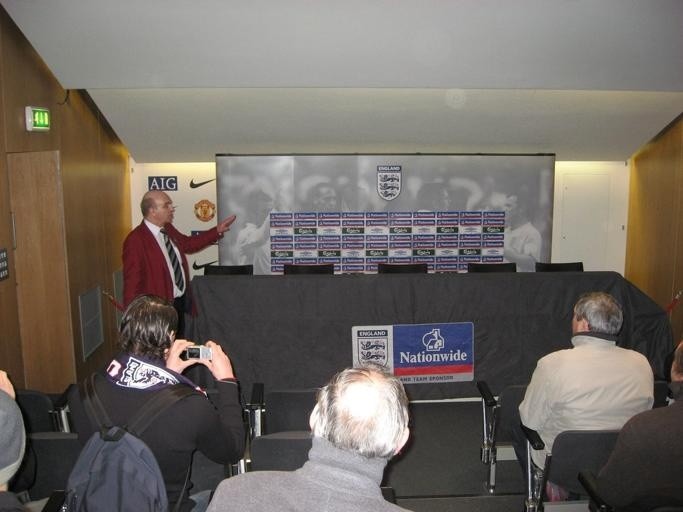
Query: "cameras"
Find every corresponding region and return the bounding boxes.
[183,346,213,363]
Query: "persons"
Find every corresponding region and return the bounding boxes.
[500,190,540,274]
[413,183,450,214]
[586,340,683,509]
[229,191,281,275]
[306,183,347,211]
[501,290,654,486]
[119,190,237,335]
[201,363,415,512]
[64,294,245,511]
[0,368,36,512]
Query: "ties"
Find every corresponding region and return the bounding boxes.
[162,229,184,292]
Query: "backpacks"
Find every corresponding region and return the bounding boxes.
[61,372,206,512]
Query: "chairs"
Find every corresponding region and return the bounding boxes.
[380,380,500,498]
[377,263,428,275]
[284,264,334,274]
[188,382,312,497]
[536,262,583,274]
[204,265,253,276]
[468,264,516,273]
[14,382,77,493]
[520,423,621,510]
